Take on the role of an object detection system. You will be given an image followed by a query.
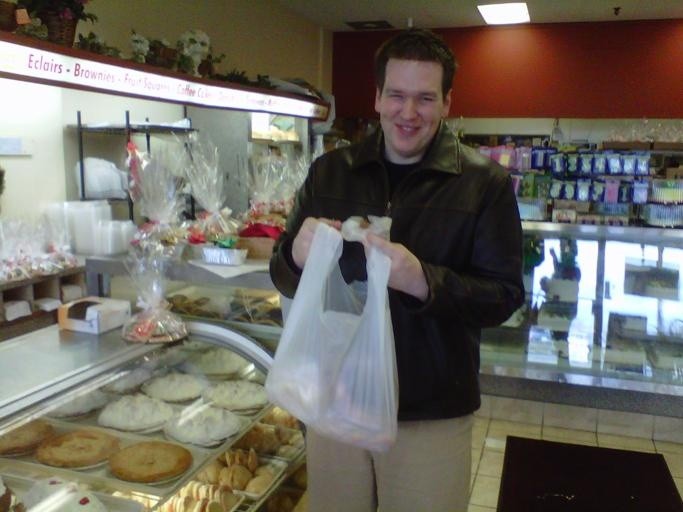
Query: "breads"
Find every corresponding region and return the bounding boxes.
[166,285,284,326]
[237,201,291,234]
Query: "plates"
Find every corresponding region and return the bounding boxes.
[130,407,180,435]
[31,442,125,471]
[1,428,58,459]
[137,453,194,487]
[56,402,110,422]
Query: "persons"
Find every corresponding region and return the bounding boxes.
[536,238,582,383]
[267,26,526,512]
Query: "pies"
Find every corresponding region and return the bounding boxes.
[0,348,306,512]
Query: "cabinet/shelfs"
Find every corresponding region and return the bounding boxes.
[477,217,680,420]
[84,244,295,357]
[4,295,309,508]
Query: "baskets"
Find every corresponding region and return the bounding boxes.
[234,237,275,260]
